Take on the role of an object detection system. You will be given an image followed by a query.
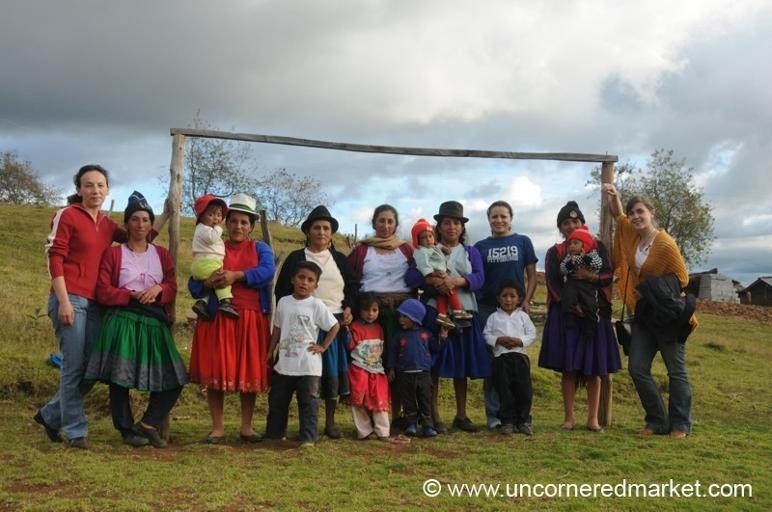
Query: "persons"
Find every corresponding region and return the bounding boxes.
[189,192,538,448]
[601,183,699,440]
[544,201,623,434]
[559,226,603,337]
[96,190,190,449]
[33,165,171,449]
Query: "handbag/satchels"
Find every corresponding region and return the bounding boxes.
[615,316,634,355]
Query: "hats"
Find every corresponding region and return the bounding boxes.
[411,219,438,248]
[567,226,593,254]
[301,206,338,235]
[396,299,427,326]
[223,193,260,221]
[434,201,469,223]
[124,191,154,224]
[195,195,227,225]
[557,201,585,227]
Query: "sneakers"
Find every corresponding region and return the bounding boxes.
[265,423,340,448]
[436,313,456,330]
[207,430,225,444]
[560,419,604,433]
[637,427,687,440]
[33,409,89,449]
[452,310,473,319]
[487,415,533,435]
[123,422,168,447]
[364,408,447,440]
[453,414,477,432]
[217,302,241,320]
[192,300,210,321]
[239,430,263,443]
